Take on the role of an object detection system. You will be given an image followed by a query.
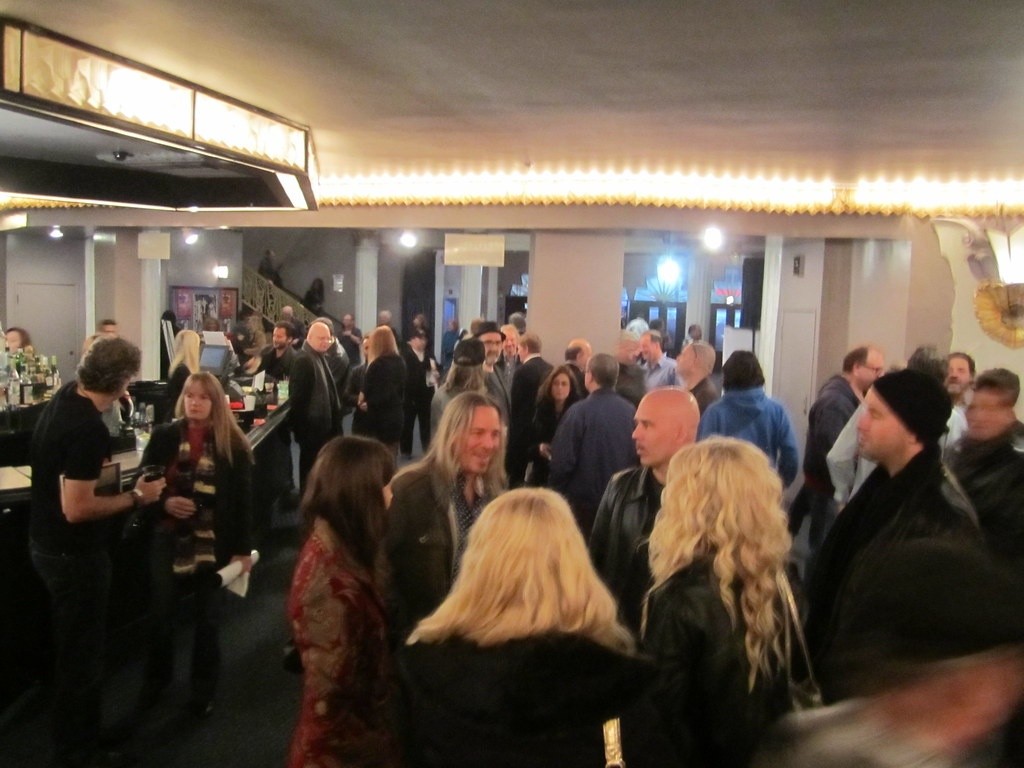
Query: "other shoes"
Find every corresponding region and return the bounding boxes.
[78,749,131,768]
[192,695,215,720]
[139,685,172,708]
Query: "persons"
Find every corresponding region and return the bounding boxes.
[258,249,276,315]
[0,310,1023,768]
[304,278,324,337]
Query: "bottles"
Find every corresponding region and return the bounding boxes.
[0,346,59,408]
[137,402,149,433]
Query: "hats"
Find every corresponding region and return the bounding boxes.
[875,369,953,445]
[472,321,507,341]
[454,338,487,366]
[410,328,428,338]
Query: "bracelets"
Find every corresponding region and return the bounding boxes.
[127,490,139,507]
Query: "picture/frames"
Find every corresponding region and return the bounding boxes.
[172,285,239,335]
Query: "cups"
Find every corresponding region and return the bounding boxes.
[143,465,166,482]
[265,383,274,392]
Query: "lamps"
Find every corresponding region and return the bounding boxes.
[182,227,200,244]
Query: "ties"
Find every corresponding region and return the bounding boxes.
[319,353,337,409]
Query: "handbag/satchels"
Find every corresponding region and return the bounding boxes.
[776,569,824,712]
